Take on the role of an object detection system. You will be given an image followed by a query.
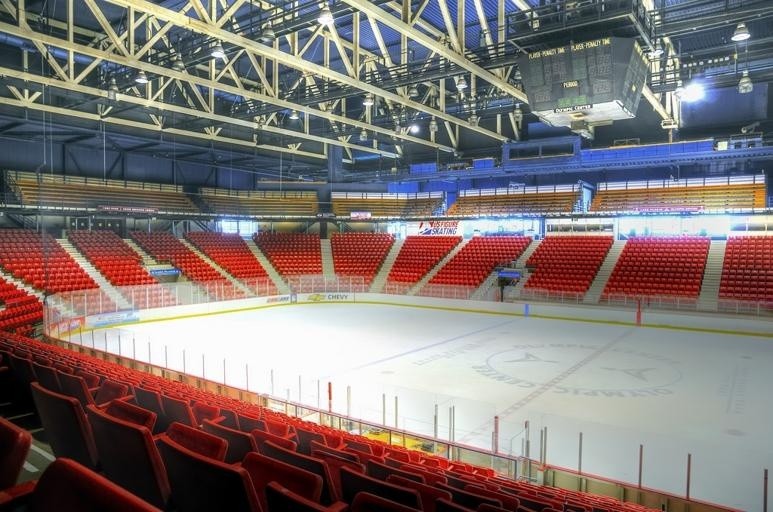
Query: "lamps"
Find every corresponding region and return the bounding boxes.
[106,1,772,121]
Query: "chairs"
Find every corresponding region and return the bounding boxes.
[0,222,252,329]
[293,416,663,511]
[0,329,293,511]
[252,230,772,311]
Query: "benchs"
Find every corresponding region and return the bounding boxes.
[4,170,197,212]
[199,187,444,214]
[444,181,766,213]
